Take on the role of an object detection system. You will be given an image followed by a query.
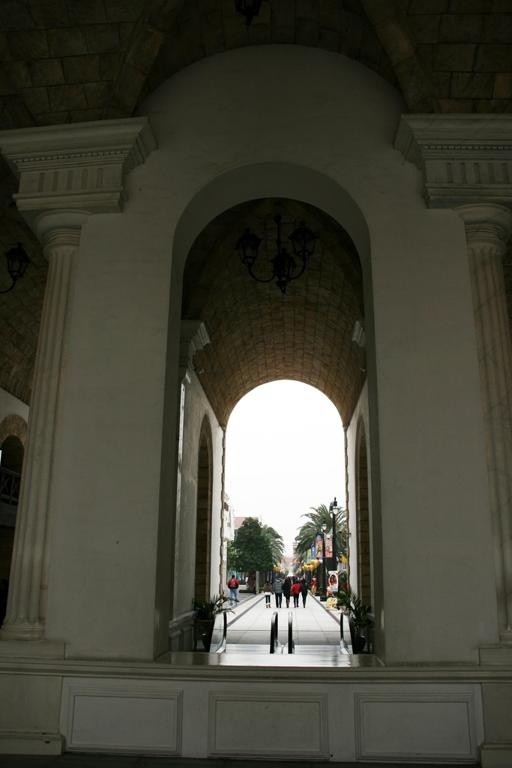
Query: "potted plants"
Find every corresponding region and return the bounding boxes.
[332,585,375,653]
[191,594,239,653]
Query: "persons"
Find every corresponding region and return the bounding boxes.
[228,575,239,602]
[263,576,309,608]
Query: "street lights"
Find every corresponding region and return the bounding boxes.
[320,517,327,601]
[330,495,339,571]
